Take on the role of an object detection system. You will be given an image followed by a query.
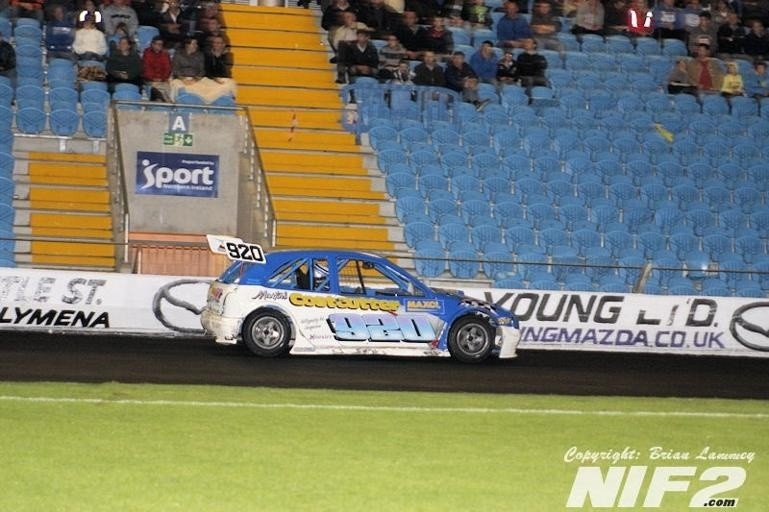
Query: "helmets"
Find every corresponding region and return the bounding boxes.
[313,263,329,284]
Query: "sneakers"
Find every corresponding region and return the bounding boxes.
[475,99,491,112]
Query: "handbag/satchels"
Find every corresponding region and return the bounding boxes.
[80,66,109,81]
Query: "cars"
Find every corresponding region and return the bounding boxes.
[200,235,522,361]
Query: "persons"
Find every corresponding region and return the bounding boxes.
[0,1,767,108]
